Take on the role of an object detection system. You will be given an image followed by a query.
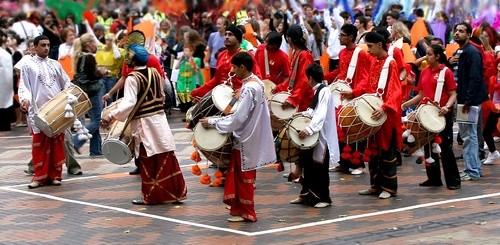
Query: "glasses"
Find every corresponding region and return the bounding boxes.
[338,33,351,37]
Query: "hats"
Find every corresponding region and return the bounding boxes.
[130,43,151,63]
[366,25,392,44]
[224,24,243,44]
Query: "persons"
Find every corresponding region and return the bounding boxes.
[0,0,500,174]
[291,62,341,207]
[18,35,71,188]
[340,32,404,199]
[102,45,187,205]
[270,24,317,184]
[401,45,461,188]
[324,23,370,176]
[448,22,486,182]
[199,52,276,222]
[102,31,167,175]
[192,25,261,187]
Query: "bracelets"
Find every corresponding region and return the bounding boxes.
[445,105,450,108]
[106,93,112,99]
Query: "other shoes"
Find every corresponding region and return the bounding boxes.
[129,168,141,175]
[11,122,29,127]
[329,165,366,175]
[132,199,144,205]
[283,171,304,184]
[73,145,81,155]
[450,183,461,190]
[24,169,35,176]
[72,169,83,176]
[227,216,247,223]
[89,152,104,158]
[456,137,500,164]
[52,179,63,186]
[418,178,441,187]
[358,188,375,195]
[314,201,331,209]
[289,197,303,205]
[27,181,44,188]
[378,191,393,199]
[459,168,479,181]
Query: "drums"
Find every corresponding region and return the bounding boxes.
[33,84,94,139]
[274,115,320,163]
[399,68,416,86]
[260,79,278,102]
[101,117,136,165]
[328,79,353,109]
[338,93,388,144]
[186,84,236,127]
[401,102,447,154]
[267,91,299,132]
[194,116,233,173]
